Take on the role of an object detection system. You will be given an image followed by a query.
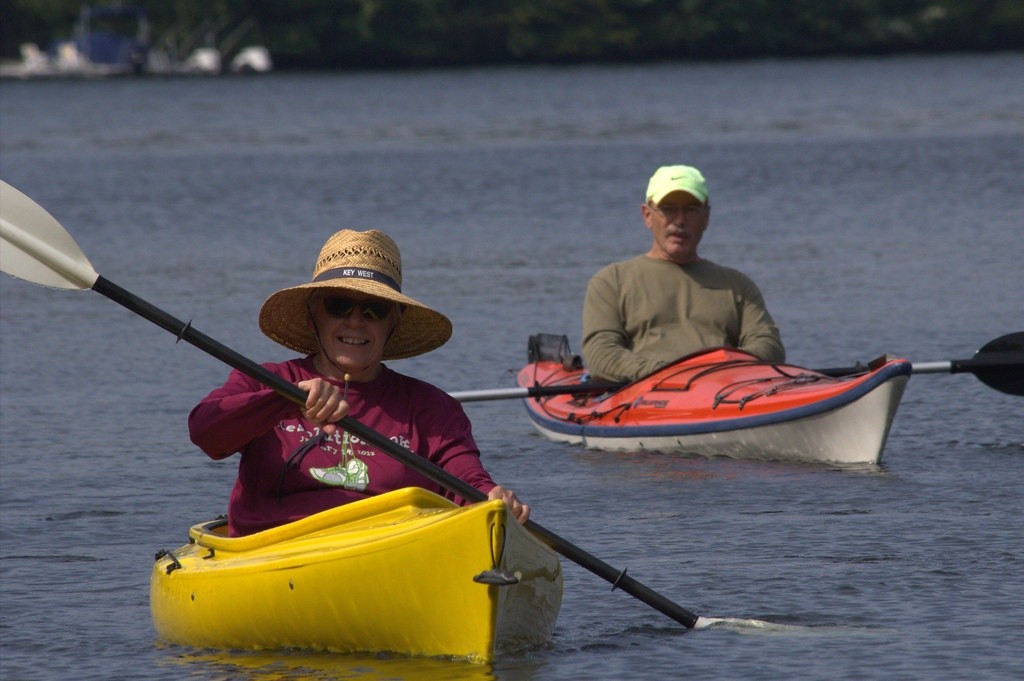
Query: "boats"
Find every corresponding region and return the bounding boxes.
[148,485,564,666]
[514,354,914,470]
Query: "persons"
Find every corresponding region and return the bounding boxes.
[581,164,785,385]
[187,229,529,537]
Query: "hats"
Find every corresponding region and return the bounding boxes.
[259,230,453,361]
[644,164,708,206]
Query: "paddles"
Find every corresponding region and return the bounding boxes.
[448,330,1024,402]
[1,180,785,628]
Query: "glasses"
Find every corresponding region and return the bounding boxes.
[314,293,394,322]
[649,202,705,219]
